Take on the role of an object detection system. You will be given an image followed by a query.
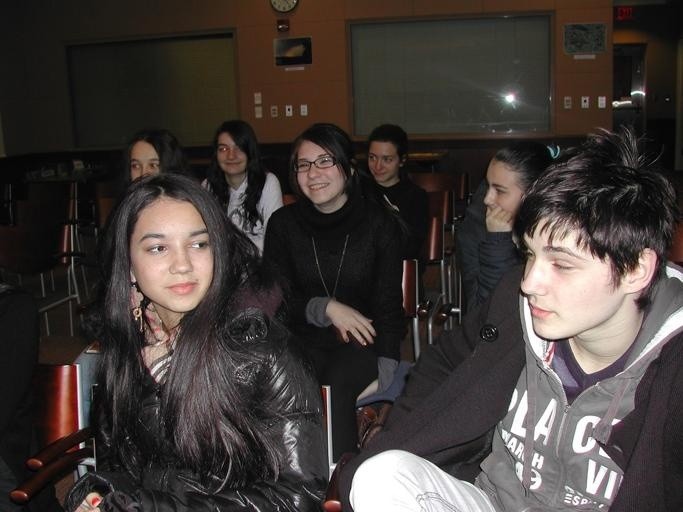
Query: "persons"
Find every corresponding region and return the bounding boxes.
[337,121,682,510]
[382,139,572,436]
[359,120,433,404]
[197,117,285,263]
[74,126,262,424]
[260,120,414,508]
[68,170,330,511]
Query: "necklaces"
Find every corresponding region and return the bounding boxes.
[311,229,351,301]
[151,305,181,356]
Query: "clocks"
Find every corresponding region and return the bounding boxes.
[268,1,298,13]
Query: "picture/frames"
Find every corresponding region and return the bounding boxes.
[560,22,607,54]
[271,36,312,68]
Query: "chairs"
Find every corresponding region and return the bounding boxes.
[11,384,356,510]
[284,171,463,361]
[1,181,91,342]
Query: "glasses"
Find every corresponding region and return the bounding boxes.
[293,155,338,172]
